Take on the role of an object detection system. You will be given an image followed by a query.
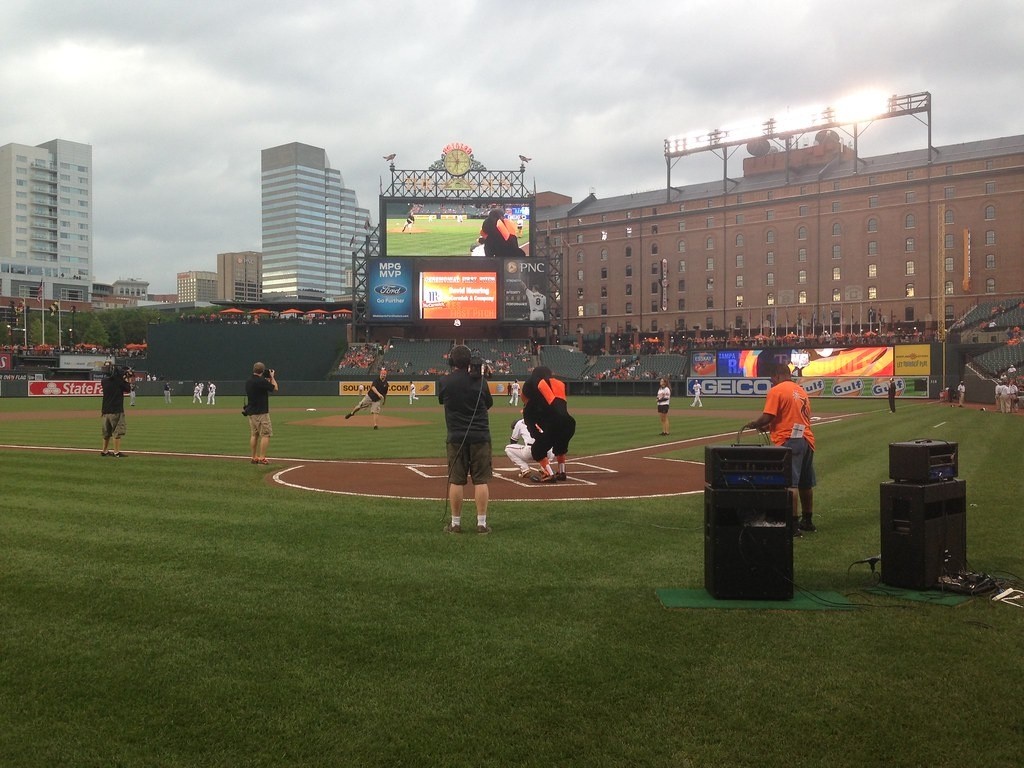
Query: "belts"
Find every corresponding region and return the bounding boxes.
[527,443,533,446]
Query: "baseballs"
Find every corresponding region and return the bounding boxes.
[390,345,393,348]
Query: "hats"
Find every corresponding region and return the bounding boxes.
[890,378,894,380]
[695,380,699,383]
[380,370,387,375]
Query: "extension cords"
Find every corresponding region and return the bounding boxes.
[992,588,1013,601]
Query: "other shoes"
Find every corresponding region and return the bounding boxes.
[659,432,669,436]
[690,404,703,407]
[477,525,492,535]
[345,412,354,419]
[792,518,816,536]
[251,456,269,464]
[889,411,893,413]
[374,424,377,430]
[518,468,531,478]
[443,523,462,535]
[540,470,566,483]
[101,451,128,458]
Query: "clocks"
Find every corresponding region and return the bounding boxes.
[446,149,469,174]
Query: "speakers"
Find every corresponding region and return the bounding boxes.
[704,485,794,600]
[880,480,966,590]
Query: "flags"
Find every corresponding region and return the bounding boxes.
[16,298,24,314]
[37,280,42,303]
[49,299,59,316]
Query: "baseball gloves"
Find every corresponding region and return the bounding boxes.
[511,418,521,429]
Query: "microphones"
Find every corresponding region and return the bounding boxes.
[856,554,881,564]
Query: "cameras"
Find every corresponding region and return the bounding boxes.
[263,369,273,378]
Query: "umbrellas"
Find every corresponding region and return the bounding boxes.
[332,309,353,315]
[306,310,328,314]
[127,343,148,350]
[864,331,877,336]
[76,343,91,350]
[280,309,303,314]
[754,334,768,339]
[643,338,660,346]
[86,344,97,348]
[784,333,797,337]
[831,332,845,337]
[247,309,271,315]
[220,308,245,314]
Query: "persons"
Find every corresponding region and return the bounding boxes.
[508,379,521,406]
[409,381,418,405]
[690,380,703,407]
[656,378,672,435]
[130,371,217,406]
[345,369,388,429]
[401,201,529,258]
[949,300,1024,413]
[245,362,278,465]
[504,405,555,478]
[101,362,131,458]
[438,346,492,533]
[600,329,938,356]
[178,310,354,325]
[339,338,545,377]
[520,280,547,320]
[748,366,817,530]
[887,378,896,414]
[523,366,577,482]
[1,342,145,361]
[583,356,683,381]
